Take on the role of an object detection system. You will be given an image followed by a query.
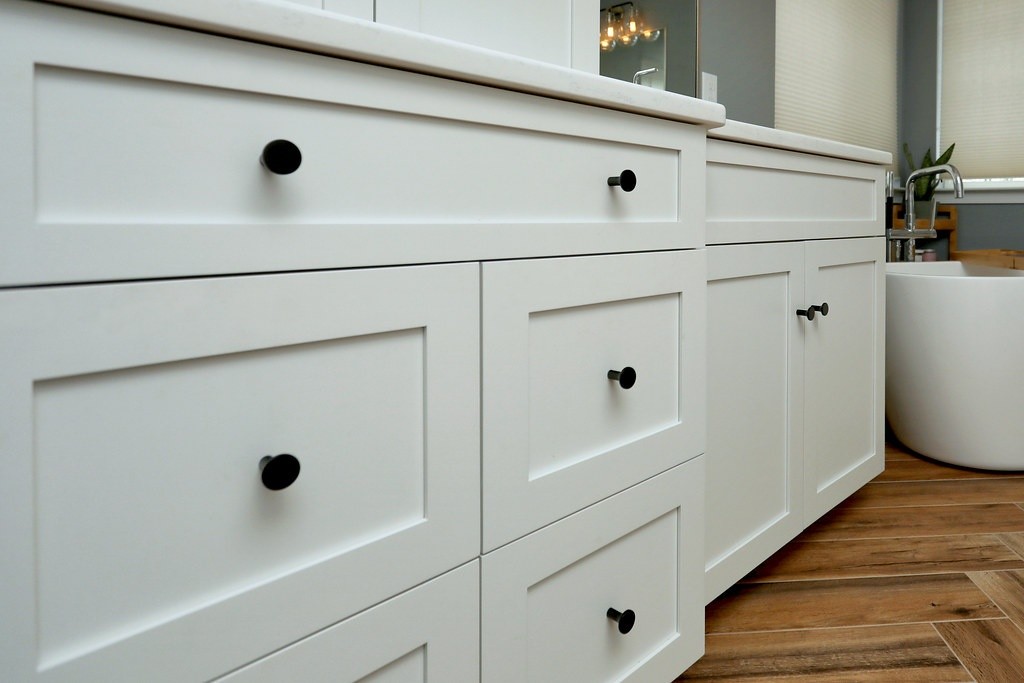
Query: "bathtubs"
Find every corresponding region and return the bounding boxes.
[885,258,1024,472]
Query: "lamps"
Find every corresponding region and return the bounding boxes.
[600,2,660,52]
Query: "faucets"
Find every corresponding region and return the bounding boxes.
[905,166,965,230]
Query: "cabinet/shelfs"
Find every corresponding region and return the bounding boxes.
[0,0,724,683]
[707,118,893,606]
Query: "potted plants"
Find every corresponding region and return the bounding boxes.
[903,141,956,218]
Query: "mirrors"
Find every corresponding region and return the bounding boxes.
[599,0,702,98]
